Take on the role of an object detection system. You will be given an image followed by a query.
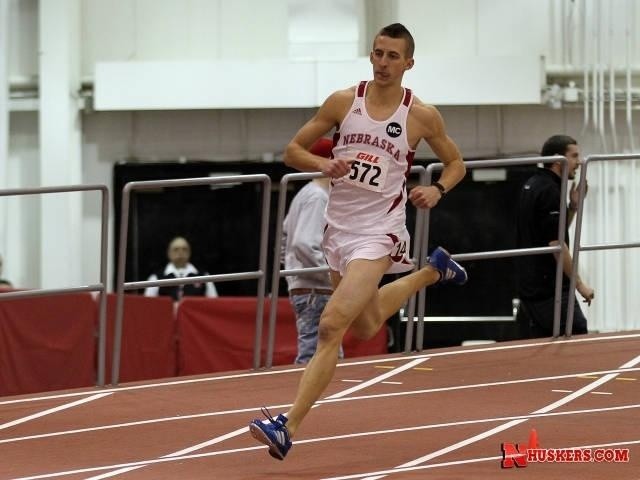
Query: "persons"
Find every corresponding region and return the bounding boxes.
[512,135,594,336]
[143,235,219,303]
[250,24,468,460]
[280,137,344,364]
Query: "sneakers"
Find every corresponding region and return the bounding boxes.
[424,246,469,286]
[249,405,293,461]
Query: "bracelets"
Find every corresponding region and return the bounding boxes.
[432,181,447,199]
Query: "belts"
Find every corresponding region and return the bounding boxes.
[288,286,333,296]
